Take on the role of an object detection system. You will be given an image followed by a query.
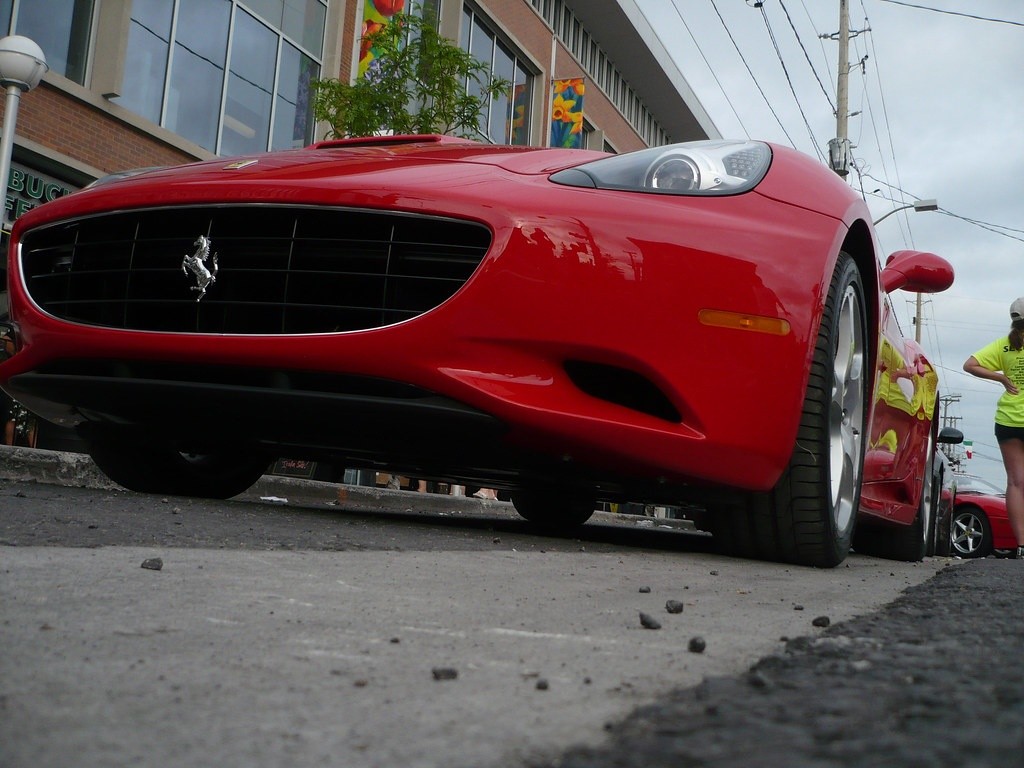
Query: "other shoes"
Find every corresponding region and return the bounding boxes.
[1015,544,1024,559]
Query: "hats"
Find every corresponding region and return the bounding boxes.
[1009,296,1024,321]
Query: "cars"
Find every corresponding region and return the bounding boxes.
[937,473,1024,561]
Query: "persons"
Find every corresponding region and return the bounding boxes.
[963,297,1024,560]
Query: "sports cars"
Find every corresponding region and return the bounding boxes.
[3,133,966,568]
